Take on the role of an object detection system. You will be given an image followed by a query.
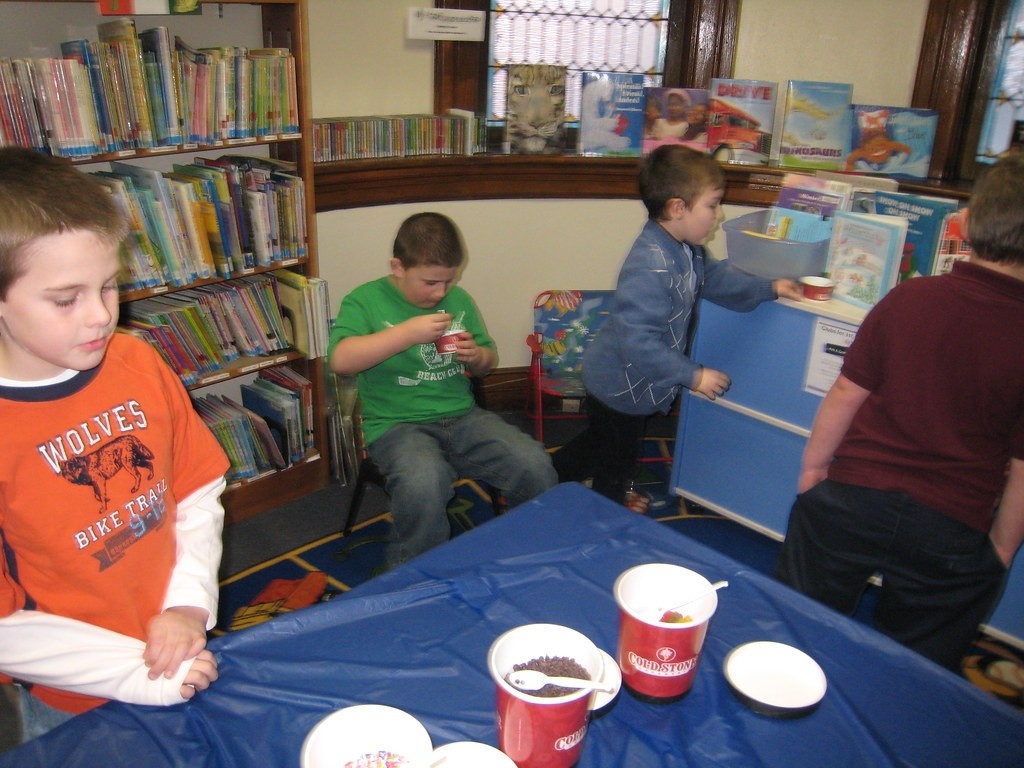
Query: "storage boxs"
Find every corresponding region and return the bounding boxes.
[721,206,832,281]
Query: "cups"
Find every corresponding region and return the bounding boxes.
[613,562,719,698]
[487,622,604,768]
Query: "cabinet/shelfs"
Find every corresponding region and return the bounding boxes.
[1,1,337,526]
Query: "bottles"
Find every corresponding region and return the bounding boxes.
[623,479,640,507]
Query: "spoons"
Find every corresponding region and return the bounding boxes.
[509,666,608,692]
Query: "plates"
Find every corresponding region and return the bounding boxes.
[299,705,520,768]
[592,648,622,711]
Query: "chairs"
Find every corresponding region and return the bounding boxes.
[341,371,483,536]
[526,287,619,443]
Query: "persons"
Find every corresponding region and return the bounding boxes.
[764,159,1024,665]
[329,213,559,576]
[647,92,707,142]
[548,141,806,505]
[0,141,231,750]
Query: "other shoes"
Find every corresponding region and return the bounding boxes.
[625,497,648,515]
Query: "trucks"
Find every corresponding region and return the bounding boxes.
[708,96,773,163]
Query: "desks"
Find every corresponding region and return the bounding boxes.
[312,146,985,211]
[0,482,1024,768]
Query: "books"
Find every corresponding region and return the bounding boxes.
[83,154,308,293]
[114,269,330,388]
[1,13,300,158]
[639,82,709,155]
[188,365,315,481]
[502,65,567,156]
[709,77,777,162]
[576,70,645,157]
[843,101,940,181]
[777,78,852,169]
[766,167,971,311]
[311,108,488,162]
[324,360,369,489]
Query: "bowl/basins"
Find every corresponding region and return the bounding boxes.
[798,275,835,302]
[433,329,468,355]
[723,640,828,716]
